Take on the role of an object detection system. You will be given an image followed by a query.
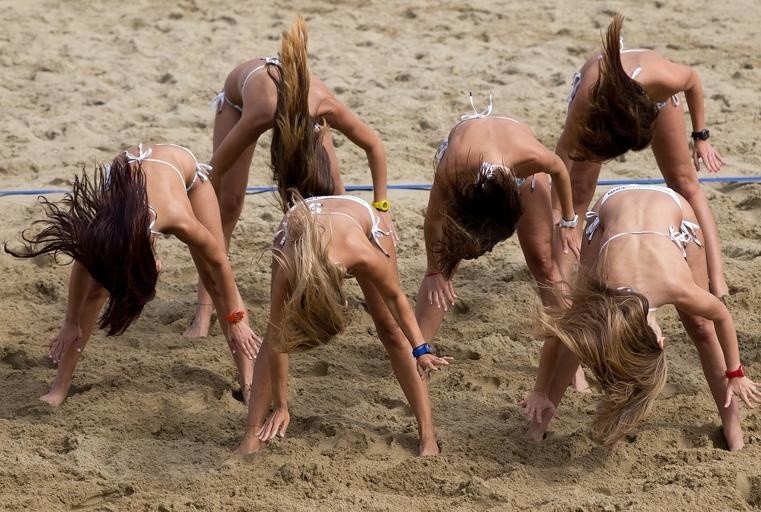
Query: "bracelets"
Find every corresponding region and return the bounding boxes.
[726,366,744,378]
[413,343,434,358]
[225,310,244,326]
[424,269,445,278]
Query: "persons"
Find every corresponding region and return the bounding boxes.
[38,142,263,408]
[234,195,454,460]
[414,115,594,394]
[550,48,733,298]
[516,184,761,454]
[183,57,402,337]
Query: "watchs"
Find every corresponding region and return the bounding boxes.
[691,130,710,141]
[371,199,391,214]
[557,214,579,229]
